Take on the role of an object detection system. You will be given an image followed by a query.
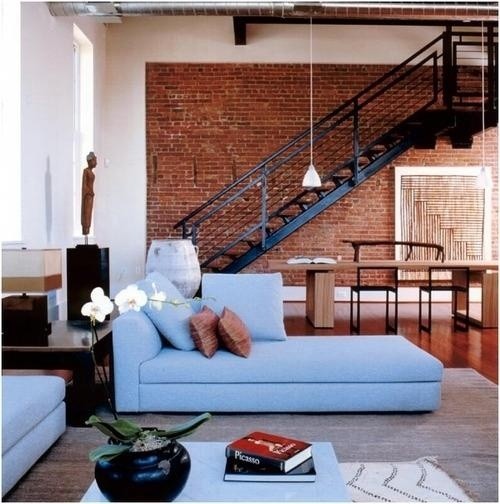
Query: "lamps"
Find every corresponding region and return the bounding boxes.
[301,17,322,187]
[2,247,62,339]
[473,19,495,191]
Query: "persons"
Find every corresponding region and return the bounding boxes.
[81,152,97,235]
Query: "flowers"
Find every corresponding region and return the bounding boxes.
[79,284,212,462]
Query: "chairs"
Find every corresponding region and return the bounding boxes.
[350,266,398,335]
[418,266,470,334]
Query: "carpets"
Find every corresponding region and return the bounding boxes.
[336,455,480,502]
[2,366,498,502]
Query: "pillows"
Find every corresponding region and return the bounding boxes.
[201,272,288,340]
[135,269,196,351]
[188,304,222,359]
[218,306,252,358]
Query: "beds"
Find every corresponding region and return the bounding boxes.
[111,297,443,414]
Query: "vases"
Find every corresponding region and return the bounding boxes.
[94,439,190,502]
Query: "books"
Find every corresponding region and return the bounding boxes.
[222,430,317,483]
[285,257,338,264]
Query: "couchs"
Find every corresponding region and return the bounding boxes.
[2,375,68,502]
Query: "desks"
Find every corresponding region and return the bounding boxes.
[2,320,114,429]
[267,260,498,328]
